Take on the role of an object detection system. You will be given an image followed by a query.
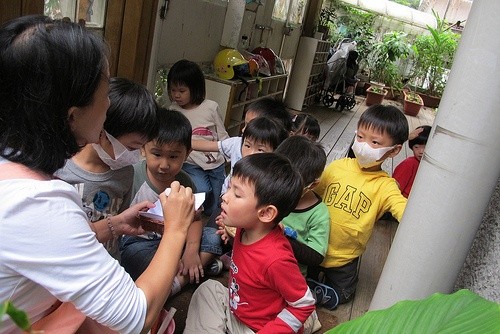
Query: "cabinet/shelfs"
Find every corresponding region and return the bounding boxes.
[288,36,332,112]
[202,72,290,135]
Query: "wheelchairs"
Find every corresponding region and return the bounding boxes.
[323,60,360,113]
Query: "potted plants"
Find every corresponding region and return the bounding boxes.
[356,7,461,117]
[314,5,338,39]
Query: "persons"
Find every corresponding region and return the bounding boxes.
[392,124,431,197]
[309,104,407,309]
[53,76,161,263]
[166,59,231,228]
[122,99,330,334]
[0,15,195,334]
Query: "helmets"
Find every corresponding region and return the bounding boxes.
[213,48,251,81]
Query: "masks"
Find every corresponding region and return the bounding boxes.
[350,130,398,169]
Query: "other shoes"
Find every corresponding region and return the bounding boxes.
[203,259,223,276]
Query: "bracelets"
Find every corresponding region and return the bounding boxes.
[107,216,118,240]
